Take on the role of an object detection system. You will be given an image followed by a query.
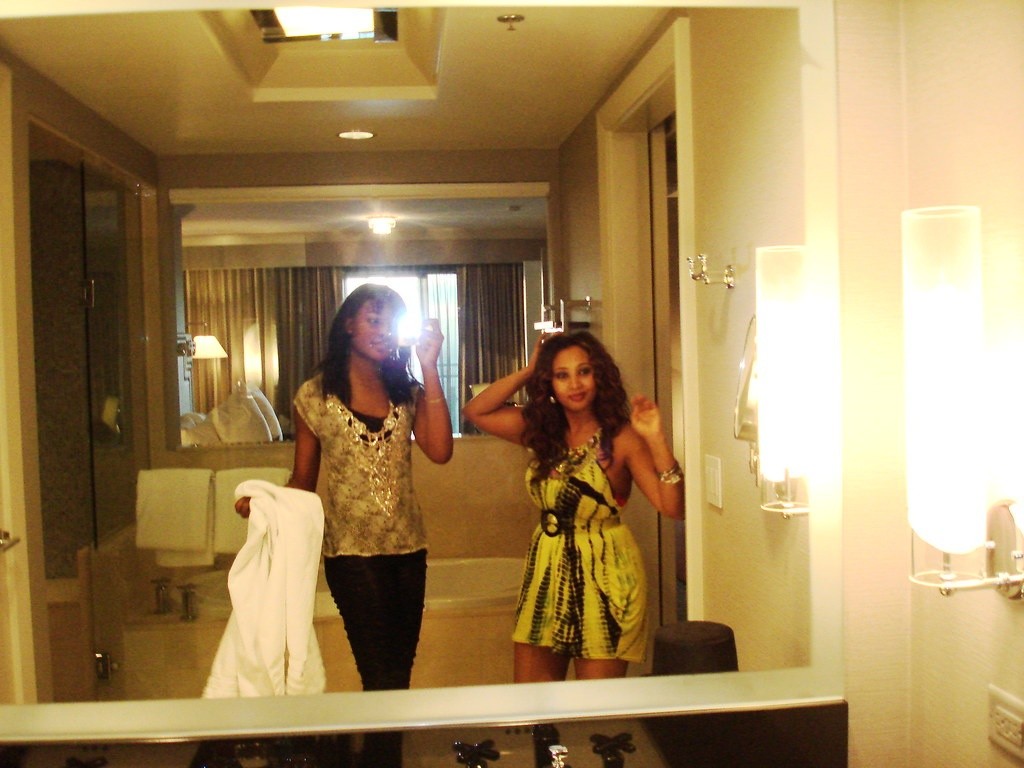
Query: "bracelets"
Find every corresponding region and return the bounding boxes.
[658,461,683,484]
[424,398,444,405]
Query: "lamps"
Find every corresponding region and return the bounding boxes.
[902,204,1024,602]
[187,320,228,360]
[368,217,396,234]
[755,246,808,518]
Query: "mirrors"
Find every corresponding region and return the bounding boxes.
[0,7,813,705]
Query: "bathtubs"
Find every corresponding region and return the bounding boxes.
[121,552,576,701]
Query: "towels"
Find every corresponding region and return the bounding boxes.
[200,480,326,699]
[136,469,214,567]
[214,468,293,553]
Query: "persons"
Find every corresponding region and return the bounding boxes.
[464,331,686,683]
[234,283,454,689]
